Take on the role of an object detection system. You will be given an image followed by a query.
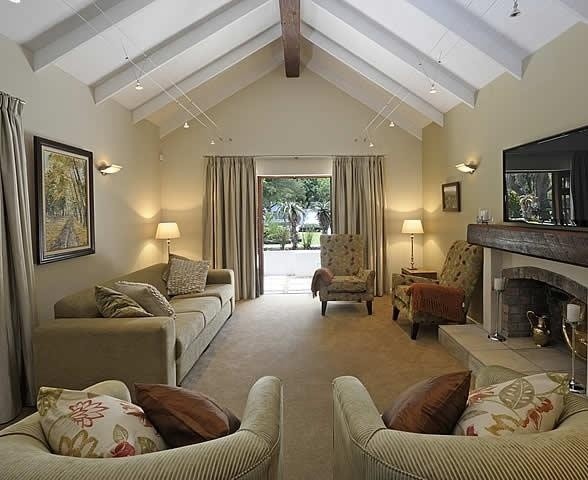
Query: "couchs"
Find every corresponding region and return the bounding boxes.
[31,264,237,407]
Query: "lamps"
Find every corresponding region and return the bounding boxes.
[455,163,476,174]
[401,220,424,271]
[154,222,182,258]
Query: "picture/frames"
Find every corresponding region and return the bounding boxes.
[442,183,461,213]
[34,135,95,265]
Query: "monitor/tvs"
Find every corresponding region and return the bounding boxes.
[502,125,588,228]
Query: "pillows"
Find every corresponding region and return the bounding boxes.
[134,382,241,447]
[95,283,153,318]
[161,253,192,281]
[382,370,472,433]
[455,373,569,436]
[166,256,210,295]
[112,281,176,317]
[37,386,166,458]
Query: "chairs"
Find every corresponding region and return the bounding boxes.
[331,366,588,480]
[0,376,284,480]
[392,240,483,339]
[319,234,376,316]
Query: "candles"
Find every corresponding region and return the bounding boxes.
[567,304,581,322]
[494,278,505,291]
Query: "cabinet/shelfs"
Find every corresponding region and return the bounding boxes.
[402,268,437,279]
